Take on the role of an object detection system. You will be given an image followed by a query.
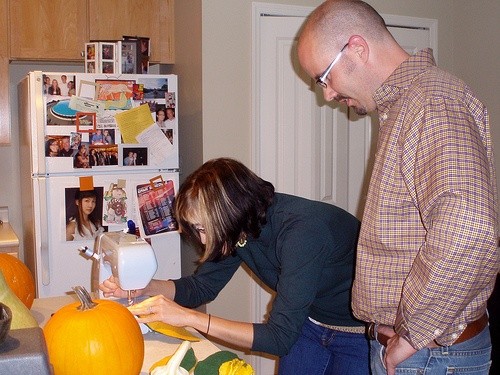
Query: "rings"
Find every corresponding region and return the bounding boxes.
[150,306,153,313]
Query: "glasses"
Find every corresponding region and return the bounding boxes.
[317,43,349,89]
[187,223,207,233]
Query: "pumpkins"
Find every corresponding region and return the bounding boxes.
[0,252,35,311]
[41,285,144,375]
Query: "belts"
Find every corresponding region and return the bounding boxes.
[365,309,489,348]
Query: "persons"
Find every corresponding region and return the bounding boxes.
[45,133,91,168]
[98,158,372,375]
[89,149,118,166]
[123,152,145,165]
[156,108,175,128]
[87,46,95,73]
[297,0,500,375]
[92,130,113,145]
[167,93,175,108]
[43,75,75,96]
[66,188,105,241]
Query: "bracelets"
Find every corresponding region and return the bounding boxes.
[207,314,211,334]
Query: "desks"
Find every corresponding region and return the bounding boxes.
[30,296,221,375]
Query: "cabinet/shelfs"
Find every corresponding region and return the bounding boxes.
[0,0,175,148]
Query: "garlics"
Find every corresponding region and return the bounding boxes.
[150,340,191,375]
